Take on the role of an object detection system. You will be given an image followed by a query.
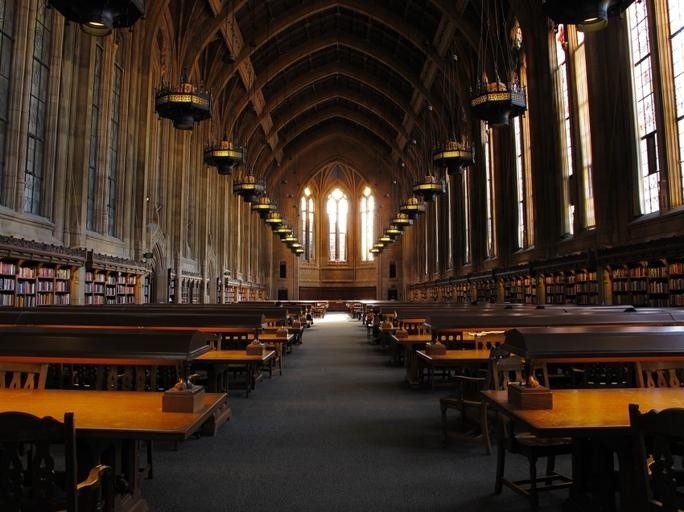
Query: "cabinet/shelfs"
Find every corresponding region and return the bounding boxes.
[405,236,684,307]
[1,235,269,309]
